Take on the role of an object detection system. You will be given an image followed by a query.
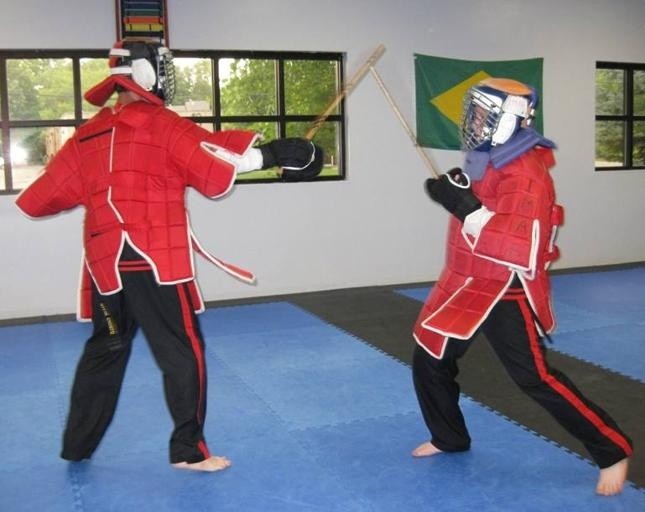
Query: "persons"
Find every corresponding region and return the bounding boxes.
[14,38,323,472]
[411,78,634,496]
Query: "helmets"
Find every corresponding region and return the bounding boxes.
[470,77,537,147]
[108,36,171,94]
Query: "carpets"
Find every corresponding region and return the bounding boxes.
[392,264,645,385]
[0,302,645,512]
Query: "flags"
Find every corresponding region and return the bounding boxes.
[415,53,544,151]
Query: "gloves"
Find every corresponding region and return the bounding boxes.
[252,137,324,181]
[425,167,482,223]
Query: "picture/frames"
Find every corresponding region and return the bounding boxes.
[115,0,170,48]
